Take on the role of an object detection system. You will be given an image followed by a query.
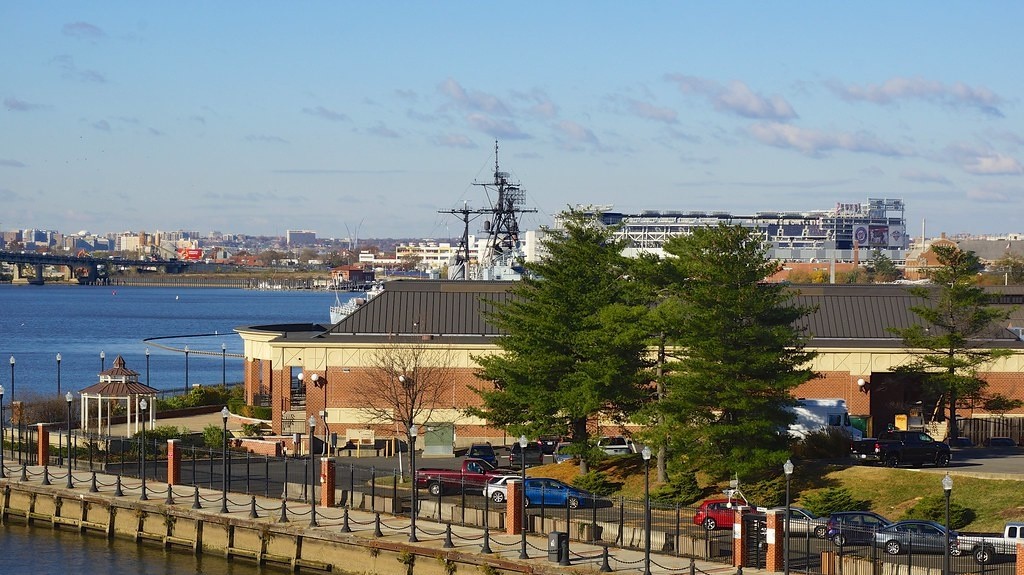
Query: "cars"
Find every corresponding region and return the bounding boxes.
[943,436,1016,447]
[539,438,576,464]
[772,506,828,540]
[524,478,583,509]
[483,475,524,502]
[694,499,757,531]
[872,520,964,557]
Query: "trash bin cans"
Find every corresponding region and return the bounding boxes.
[548,532,568,562]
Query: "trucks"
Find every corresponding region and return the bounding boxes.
[781,397,862,446]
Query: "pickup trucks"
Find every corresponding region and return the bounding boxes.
[598,436,632,455]
[416,458,514,496]
[957,523,1024,565]
[850,430,952,468]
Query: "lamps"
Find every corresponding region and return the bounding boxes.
[311,374,319,387]
[298,373,306,389]
[400,375,405,386]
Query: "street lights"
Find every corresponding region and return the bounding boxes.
[0,385,147,500]
[145,342,226,395]
[220,406,530,559]
[10,349,104,410]
[642,445,953,575]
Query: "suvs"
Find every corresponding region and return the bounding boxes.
[828,512,894,547]
[466,442,500,467]
[506,442,543,469]
